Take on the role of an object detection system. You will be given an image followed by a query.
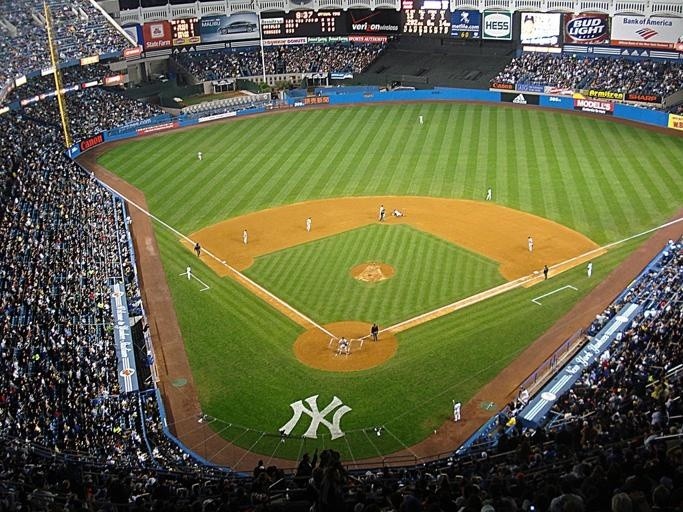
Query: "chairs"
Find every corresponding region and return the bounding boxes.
[157,412,524,512]
[1,145,160,511]
[168,39,385,83]
[522,235,683,512]
[490,49,683,110]
[1,1,164,150]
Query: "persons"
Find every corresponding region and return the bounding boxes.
[2,0,682,512]
